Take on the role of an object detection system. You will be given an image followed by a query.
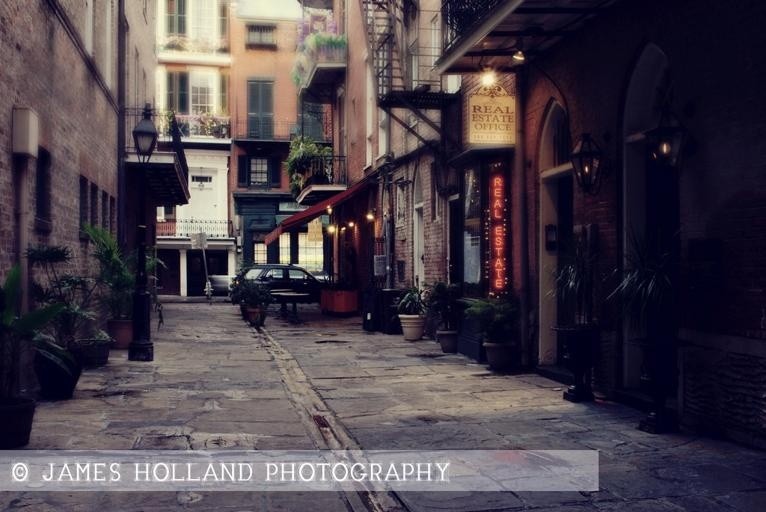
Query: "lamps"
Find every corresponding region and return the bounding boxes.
[569,104,696,200]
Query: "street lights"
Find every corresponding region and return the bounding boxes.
[124,98,160,363]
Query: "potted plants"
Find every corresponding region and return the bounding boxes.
[230,279,273,327]
[1,220,166,451]
[396,274,526,378]
[544,220,714,436]
[284,134,334,198]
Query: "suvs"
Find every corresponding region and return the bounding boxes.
[229,262,321,307]
